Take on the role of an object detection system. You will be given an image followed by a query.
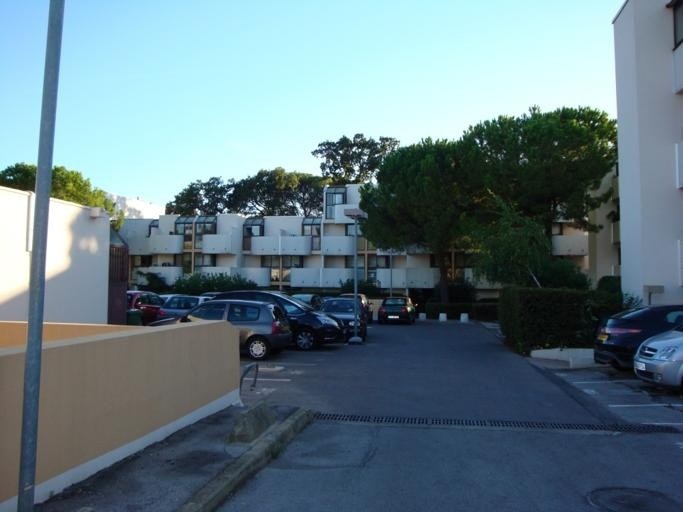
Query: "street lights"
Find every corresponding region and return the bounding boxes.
[344,209,367,346]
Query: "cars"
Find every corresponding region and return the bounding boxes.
[594,304,683,373]
[125,289,418,342]
[634,325,682,387]
[208,290,340,351]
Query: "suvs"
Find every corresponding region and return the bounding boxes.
[145,300,290,359]
